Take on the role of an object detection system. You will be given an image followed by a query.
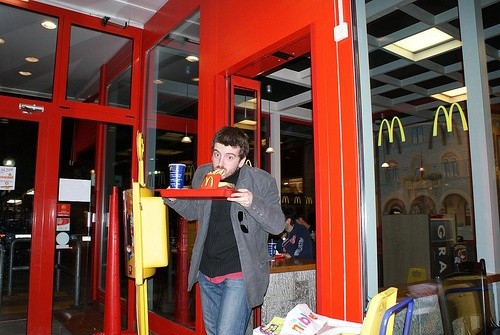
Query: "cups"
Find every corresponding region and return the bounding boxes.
[168,164,187,188]
[267,243,278,261]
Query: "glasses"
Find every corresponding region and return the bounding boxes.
[238,211,249,233]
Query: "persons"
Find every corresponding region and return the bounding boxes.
[276,209,316,259]
[163,126,285,335]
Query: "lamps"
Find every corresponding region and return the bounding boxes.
[265,86,275,153]
[181,66,192,143]
[381,113,390,168]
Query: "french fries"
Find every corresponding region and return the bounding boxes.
[207,168,224,174]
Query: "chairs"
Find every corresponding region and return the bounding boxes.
[436,259,500,335]
[361,287,415,335]
